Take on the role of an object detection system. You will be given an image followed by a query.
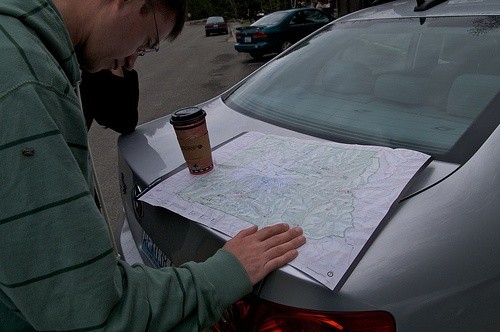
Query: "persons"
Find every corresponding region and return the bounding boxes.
[0,0,306,332]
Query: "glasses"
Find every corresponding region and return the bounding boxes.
[139,0,160,56]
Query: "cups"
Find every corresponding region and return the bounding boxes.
[170,106,214,174]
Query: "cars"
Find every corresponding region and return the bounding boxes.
[117,0,500,332]
[205,16,228,37]
[234,7,337,59]
